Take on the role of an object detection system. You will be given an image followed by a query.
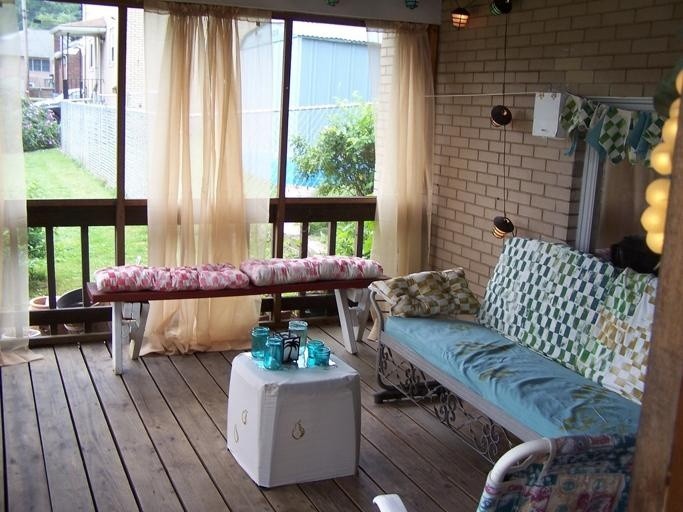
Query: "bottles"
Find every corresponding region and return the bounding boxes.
[288,320,307,355]
[251,326,283,370]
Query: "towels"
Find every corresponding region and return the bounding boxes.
[558,95,665,168]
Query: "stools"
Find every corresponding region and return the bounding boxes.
[225,344,361,489]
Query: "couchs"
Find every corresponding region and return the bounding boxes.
[369,235,660,466]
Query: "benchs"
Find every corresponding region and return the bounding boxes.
[86,275,392,375]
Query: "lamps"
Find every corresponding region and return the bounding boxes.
[489,105,512,127]
[491,216,514,239]
[449,8,469,30]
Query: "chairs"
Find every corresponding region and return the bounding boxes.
[371,434,637,511]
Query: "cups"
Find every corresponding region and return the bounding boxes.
[306,340,330,366]
